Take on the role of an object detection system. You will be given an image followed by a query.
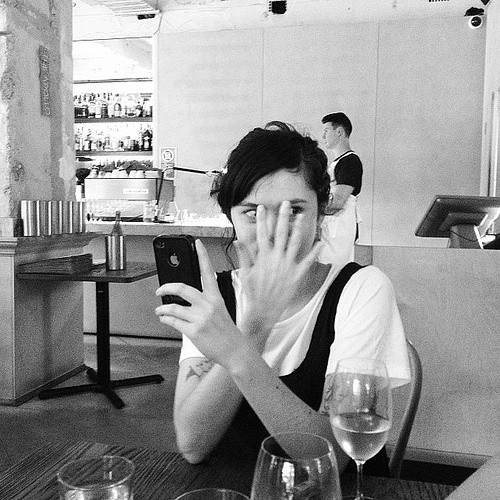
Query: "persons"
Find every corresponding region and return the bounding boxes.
[314,113,364,267]
[153,121,412,482]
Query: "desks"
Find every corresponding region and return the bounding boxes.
[17,261,164,410]
[0,440,458,500]
[82,215,238,338]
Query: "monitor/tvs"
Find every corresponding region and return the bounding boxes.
[414,194,500,250]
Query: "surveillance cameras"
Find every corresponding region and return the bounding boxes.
[468,14,483,29]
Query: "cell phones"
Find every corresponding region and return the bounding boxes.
[153,234,203,307]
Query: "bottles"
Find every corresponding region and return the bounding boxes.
[76,160,145,198]
[111,212,123,236]
[72,91,152,119]
[72,126,153,151]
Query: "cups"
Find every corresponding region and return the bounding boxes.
[252,433,343,500]
[173,488,249,500]
[142,199,156,223]
[55,457,134,499]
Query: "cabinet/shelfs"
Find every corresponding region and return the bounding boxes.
[73,117,153,156]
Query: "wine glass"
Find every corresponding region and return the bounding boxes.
[329,356,392,500]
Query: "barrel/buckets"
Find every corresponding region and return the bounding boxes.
[73,201,86,234]
[39,200,51,236]
[105,235,127,270]
[62,200,73,234]
[20,200,40,236]
[51,200,62,236]
